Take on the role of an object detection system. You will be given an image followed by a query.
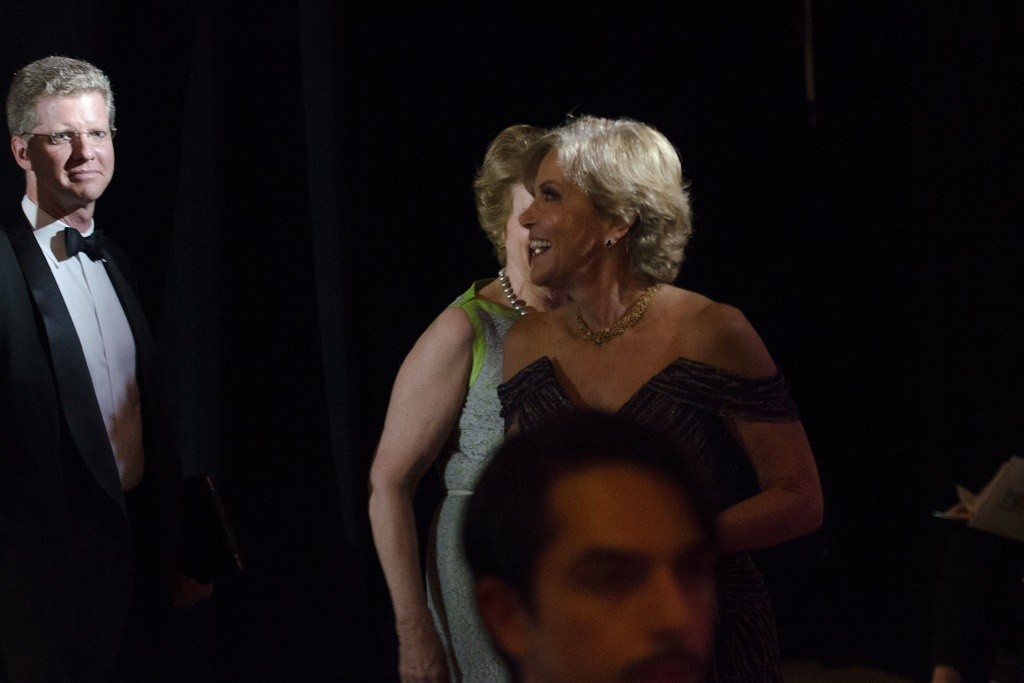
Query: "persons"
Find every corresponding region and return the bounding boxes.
[368,123,550,683]
[461,411,717,683]
[496,112,824,683]
[919,438,1024,683]
[1,55,218,683]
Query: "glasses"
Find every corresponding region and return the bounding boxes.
[25,126,117,147]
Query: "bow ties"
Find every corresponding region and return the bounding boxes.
[63,226,105,262]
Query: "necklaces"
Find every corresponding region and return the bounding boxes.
[566,281,661,345]
[497,267,528,319]
